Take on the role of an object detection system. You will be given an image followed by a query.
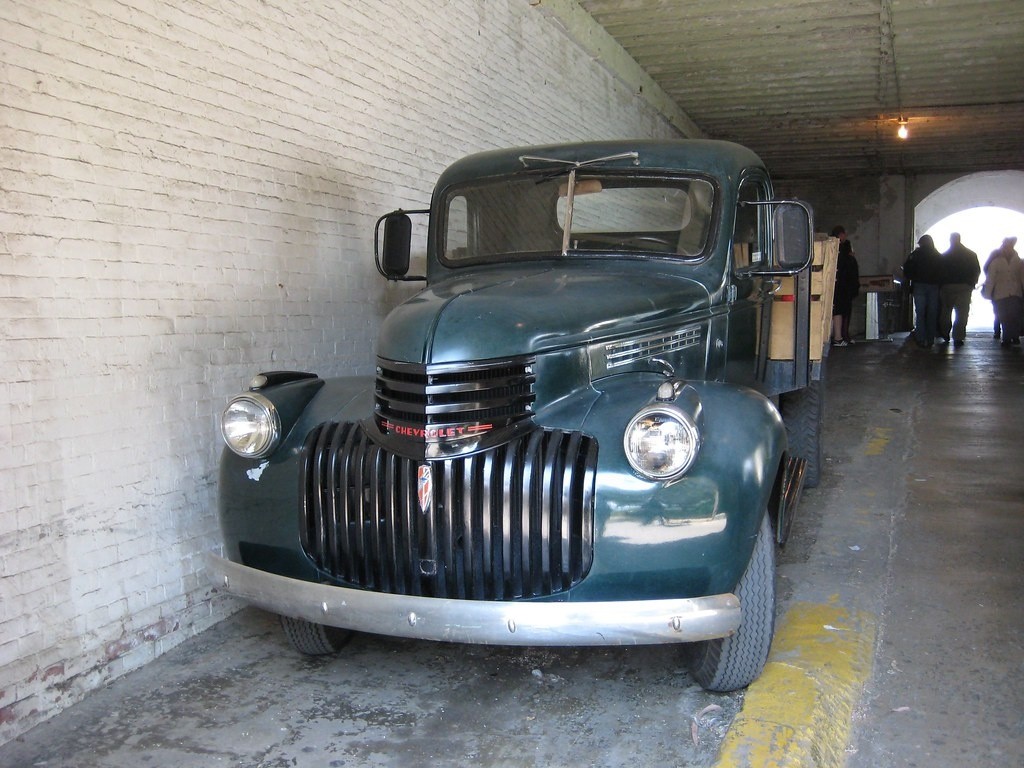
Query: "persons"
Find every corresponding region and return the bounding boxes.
[902,236,1024,347]
[829,226,863,347]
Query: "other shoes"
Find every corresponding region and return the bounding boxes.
[833,338,854,346]
[1013,340,1020,345]
[994,333,1000,339]
[954,340,964,347]
[1000,340,1011,347]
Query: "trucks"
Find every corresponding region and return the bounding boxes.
[199,135,833,696]
